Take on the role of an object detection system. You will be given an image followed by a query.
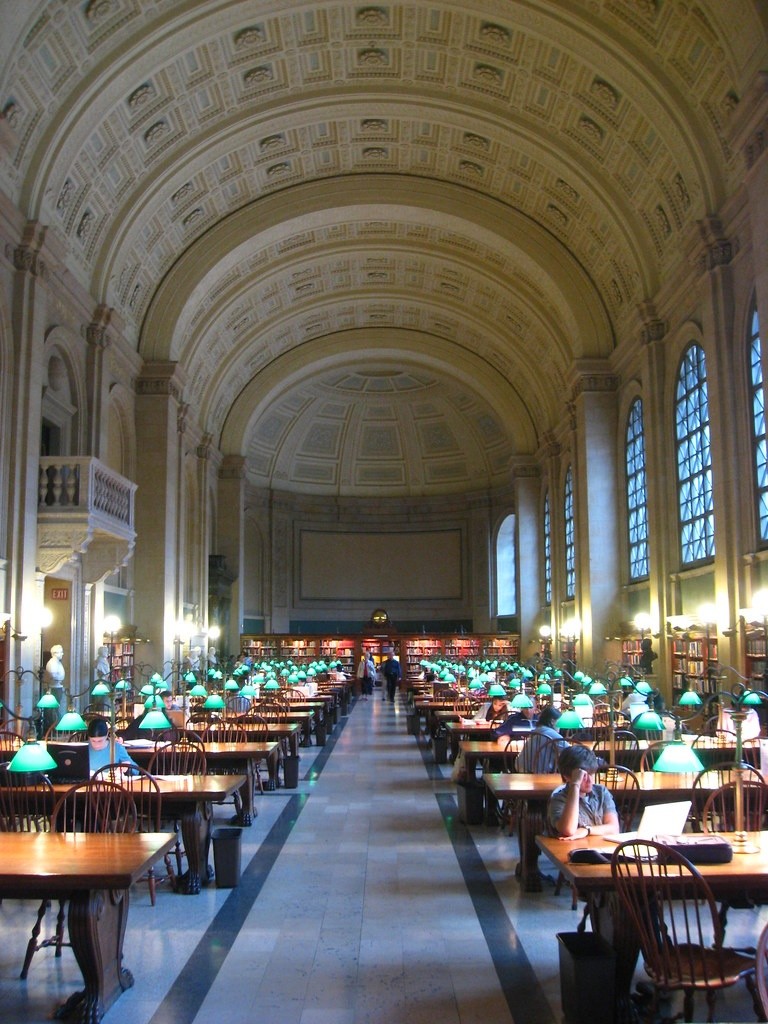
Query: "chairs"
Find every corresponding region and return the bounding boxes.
[402,669,768,1023]
[0,673,357,982]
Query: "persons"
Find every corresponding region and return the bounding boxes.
[627,692,688,740]
[514,707,607,773]
[621,680,649,714]
[58,719,139,832]
[380,652,402,702]
[458,696,520,774]
[94,647,111,678]
[527,652,540,666]
[188,647,201,668]
[357,653,377,700]
[46,646,65,683]
[638,638,658,666]
[118,651,351,768]
[549,745,672,977]
[489,698,539,771]
[207,646,216,663]
[419,660,568,711]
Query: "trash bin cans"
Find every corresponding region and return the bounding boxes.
[212,826,243,888]
[556,932,619,1024]
[406,713,419,735]
[316,688,355,744]
[283,755,300,787]
[459,777,483,823]
[430,736,447,762]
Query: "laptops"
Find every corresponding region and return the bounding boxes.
[166,710,184,727]
[602,800,693,845]
[44,742,90,786]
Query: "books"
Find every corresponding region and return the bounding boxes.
[105,641,134,702]
[242,640,520,673]
[542,625,768,723]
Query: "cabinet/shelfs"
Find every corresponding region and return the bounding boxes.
[540,639,579,663]
[103,624,138,702]
[240,635,522,687]
[597,635,647,688]
[735,632,767,693]
[666,637,720,700]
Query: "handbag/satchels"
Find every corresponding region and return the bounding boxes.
[651,834,733,865]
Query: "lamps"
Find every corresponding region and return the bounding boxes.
[0,607,341,785]
[420,607,767,796]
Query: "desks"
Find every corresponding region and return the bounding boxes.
[121,693,335,735]
[54,723,303,788]
[483,765,766,895]
[526,830,766,1023]
[414,690,593,749]
[459,733,766,825]
[0,830,179,1024]
[2,773,247,894]
[77,710,317,763]
[158,684,350,717]
[0,738,279,826]
[443,719,683,811]
[122,701,326,748]
[421,708,618,760]
